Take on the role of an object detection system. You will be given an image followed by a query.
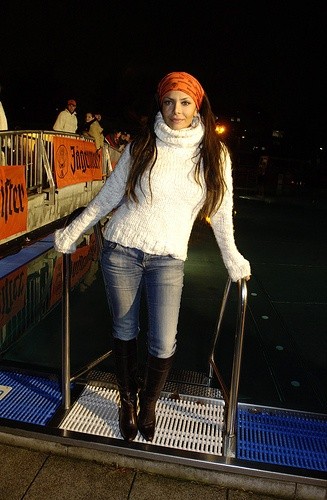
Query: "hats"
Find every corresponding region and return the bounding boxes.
[157,72,204,110]
[68,99,76,105]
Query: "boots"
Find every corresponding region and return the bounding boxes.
[111,337,172,442]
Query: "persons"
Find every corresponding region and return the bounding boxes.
[0,102,10,152]
[53,100,78,133]
[79,111,134,152]
[54,72,250,442]
